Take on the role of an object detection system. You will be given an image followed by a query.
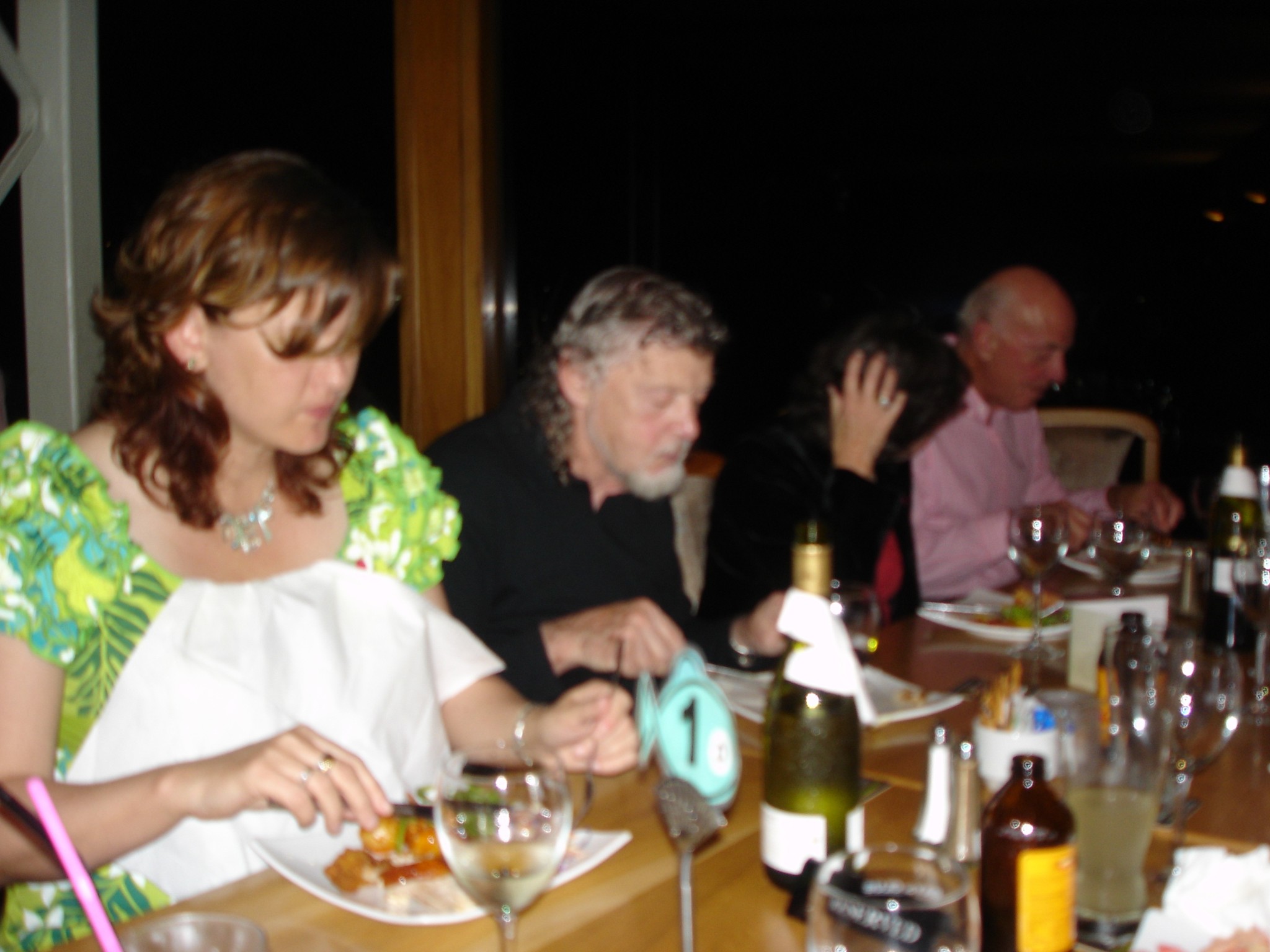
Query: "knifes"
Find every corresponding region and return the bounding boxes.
[392,802,505,817]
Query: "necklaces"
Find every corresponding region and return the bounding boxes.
[218,477,276,554]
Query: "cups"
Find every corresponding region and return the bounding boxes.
[117,910,269,952]
[807,841,981,952]
[1035,687,1166,952]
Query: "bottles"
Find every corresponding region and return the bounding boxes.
[941,740,977,860]
[980,755,1079,952]
[757,513,865,892]
[908,726,957,845]
[1203,442,1260,656]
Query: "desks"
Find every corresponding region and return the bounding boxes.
[50,538,1270,952]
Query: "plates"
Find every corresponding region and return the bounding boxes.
[231,781,634,926]
[712,661,966,728]
[915,587,1076,643]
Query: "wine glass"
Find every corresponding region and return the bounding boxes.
[1102,622,1244,882]
[1084,508,1152,598]
[1233,534,1270,726]
[433,740,573,952]
[1007,504,1071,662]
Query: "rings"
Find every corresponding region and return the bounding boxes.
[299,766,313,785]
[321,754,334,774]
[879,396,890,406]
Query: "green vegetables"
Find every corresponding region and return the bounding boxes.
[398,783,503,838]
[1001,606,1071,627]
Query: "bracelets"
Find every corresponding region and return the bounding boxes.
[728,615,754,658]
[513,701,540,769]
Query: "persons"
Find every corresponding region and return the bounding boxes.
[696,298,974,622]
[912,266,1186,598]
[420,259,789,716]
[0,149,633,952]
